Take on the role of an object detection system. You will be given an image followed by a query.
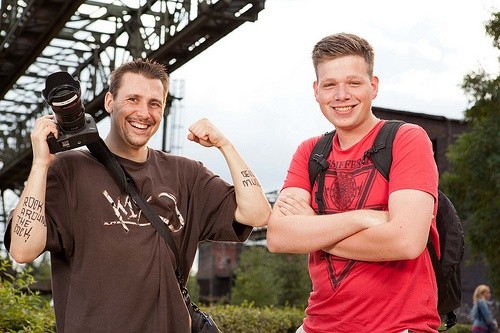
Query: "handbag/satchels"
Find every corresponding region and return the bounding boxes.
[178,296,221,333]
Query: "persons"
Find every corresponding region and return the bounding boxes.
[2,57,272,332]
[471,284,499,332]
[266,31,443,332]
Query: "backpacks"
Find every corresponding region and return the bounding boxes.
[309,120,464,333]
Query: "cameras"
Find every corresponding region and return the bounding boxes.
[42,72,99,154]
